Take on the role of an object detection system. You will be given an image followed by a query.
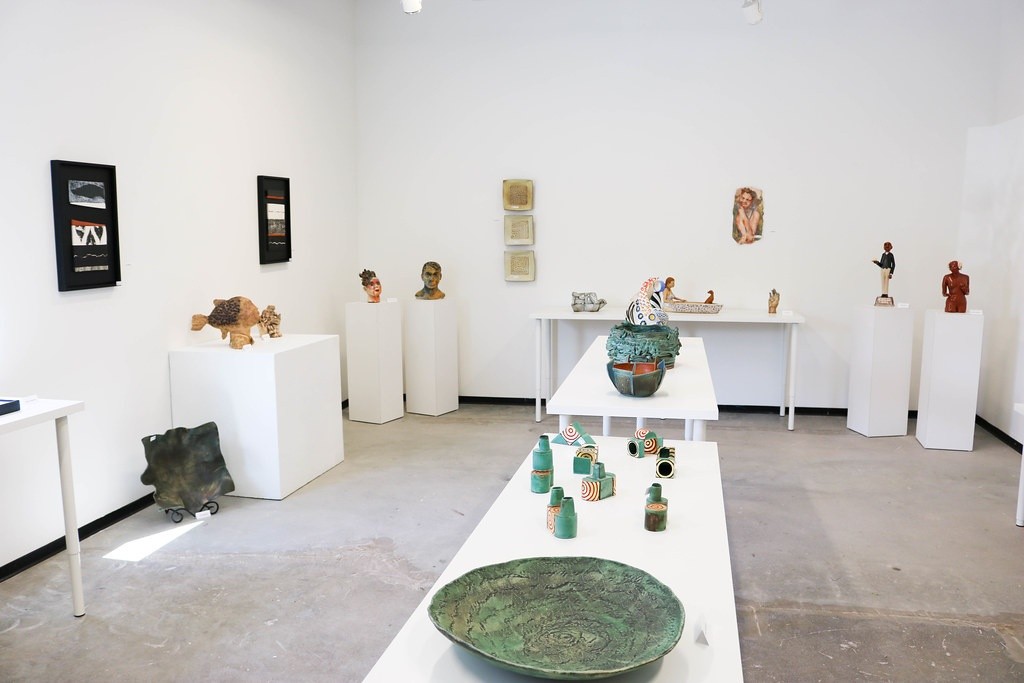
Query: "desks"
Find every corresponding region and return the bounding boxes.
[546,337,718,440]
[522,295,805,431]
[0,396,86,617]
[359,433,744,683]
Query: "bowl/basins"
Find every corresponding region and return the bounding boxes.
[608,359,667,398]
[427,555,686,681]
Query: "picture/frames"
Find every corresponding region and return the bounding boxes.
[257,175,291,266]
[50,159,121,292]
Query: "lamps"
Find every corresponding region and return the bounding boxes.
[399,0,422,14]
[742,0,764,25]
